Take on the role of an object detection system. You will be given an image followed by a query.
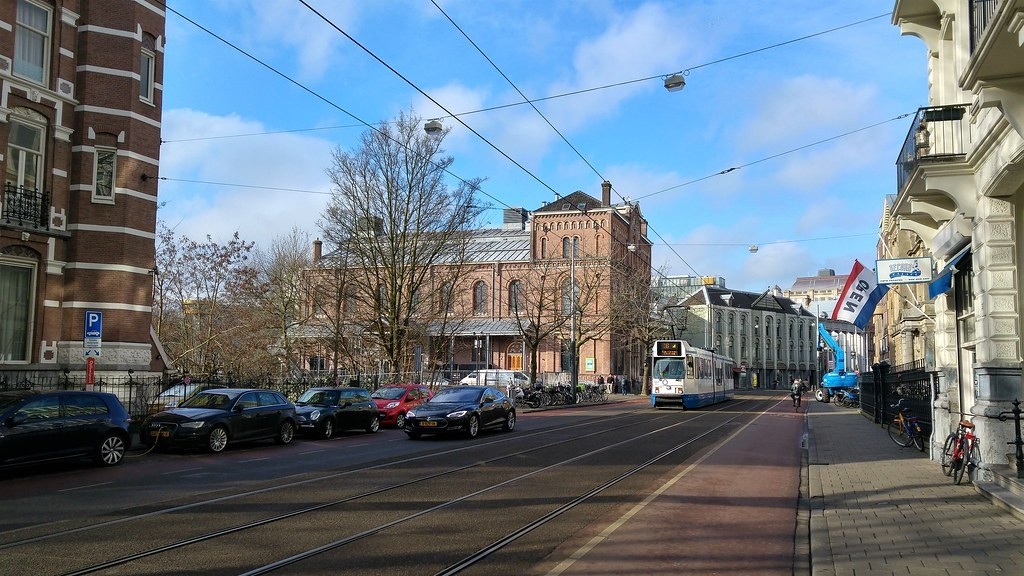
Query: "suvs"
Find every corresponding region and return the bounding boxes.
[0,390,132,481]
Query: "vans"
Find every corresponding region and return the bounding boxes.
[458,369,531,387]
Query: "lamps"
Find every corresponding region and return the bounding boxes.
[627,245,636,251]
[748,244,758,253]
[664,74,686,92]
[424,120,442,134]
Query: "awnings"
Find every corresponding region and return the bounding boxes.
[927,247,970,300]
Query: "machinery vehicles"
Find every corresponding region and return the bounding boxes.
[814,322,858,402]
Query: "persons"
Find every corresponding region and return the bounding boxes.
[597,373,628,395]
[790,379,807,407]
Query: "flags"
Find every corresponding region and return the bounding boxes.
[831,259,891,331]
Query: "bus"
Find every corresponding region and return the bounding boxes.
[651,305,735,410]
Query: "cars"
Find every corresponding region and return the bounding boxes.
[369,384,433,429]
[140,388,298,454]
[294,386,382,440]
[149,381,231,418]
[403,385,517,439]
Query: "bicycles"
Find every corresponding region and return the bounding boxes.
[513,381,609,408]
[832,393,859,408]
[792,393,801,412]
[940,409,981,486]
[887,398,926,452]
